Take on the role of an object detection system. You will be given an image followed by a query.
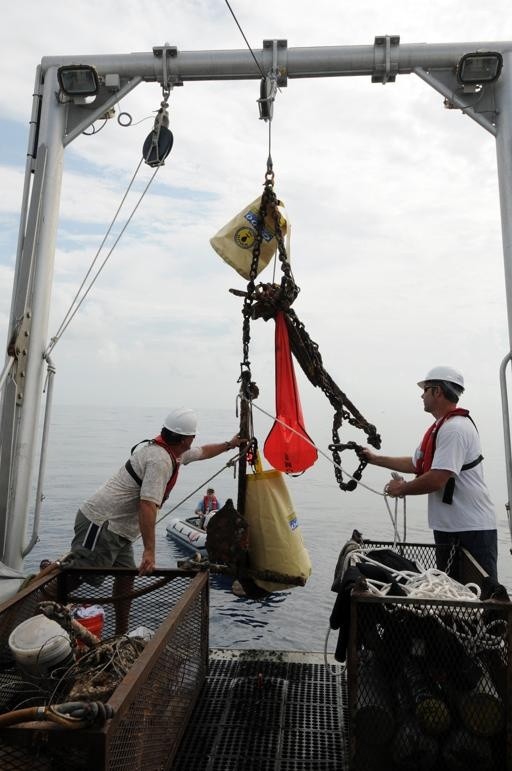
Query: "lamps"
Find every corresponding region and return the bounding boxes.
[55,65,101,105]
[456,52,502,95]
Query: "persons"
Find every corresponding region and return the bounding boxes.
[37,406,249,638]
[195,487,219,519]
[354,366,499,584]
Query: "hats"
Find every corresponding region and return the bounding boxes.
[207,489,214,492]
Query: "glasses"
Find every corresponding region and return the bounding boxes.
[424,385,438,392]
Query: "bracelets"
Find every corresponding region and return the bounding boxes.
[224,442,231,452]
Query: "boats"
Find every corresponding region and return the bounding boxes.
[166,516,209,559]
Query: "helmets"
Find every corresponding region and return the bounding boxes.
[164,408,201,436]
[416,365,465,390]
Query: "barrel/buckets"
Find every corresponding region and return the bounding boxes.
[8,612,75,675]
[72,602,105,648]
